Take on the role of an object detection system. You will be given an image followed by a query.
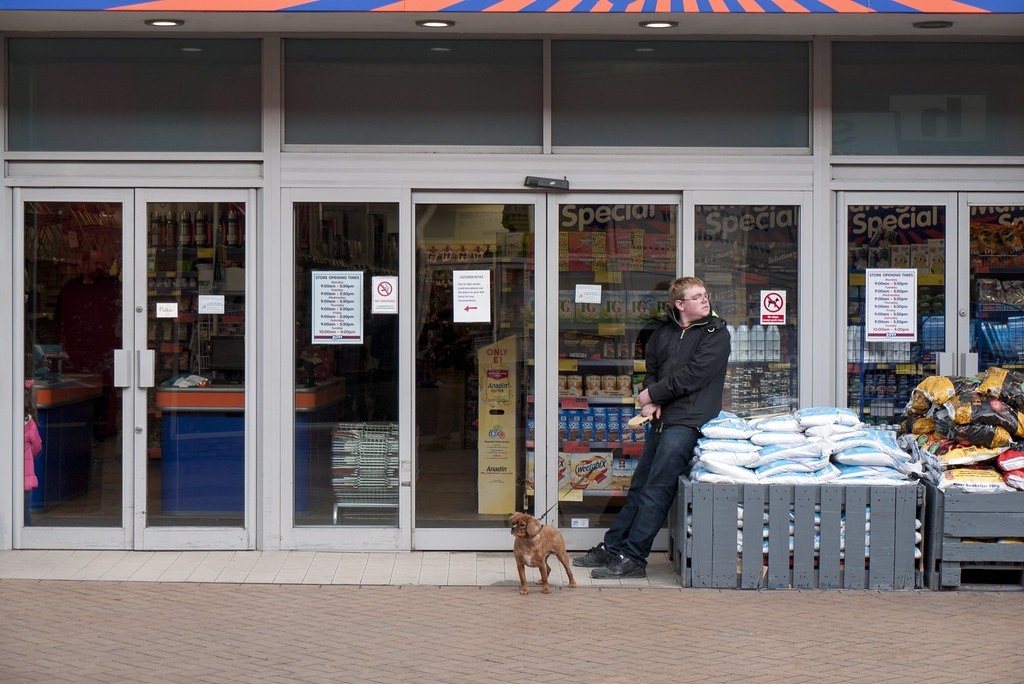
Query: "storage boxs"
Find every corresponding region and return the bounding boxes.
[525,450,639,500]
[526,405,653,443]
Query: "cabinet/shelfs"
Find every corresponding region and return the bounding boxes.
[519,321,659,516]
[116,244,245,460]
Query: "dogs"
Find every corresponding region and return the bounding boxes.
[509,512,576,595]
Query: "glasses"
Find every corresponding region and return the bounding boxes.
[677,293,711,302]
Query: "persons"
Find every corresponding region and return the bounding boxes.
[51,248,121,446]
[571,277,730,579]
[427,323,476,451]
[335,313,399,425]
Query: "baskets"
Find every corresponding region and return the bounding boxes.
[328,422,420,518]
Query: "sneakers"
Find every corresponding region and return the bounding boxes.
[591,553,648,579]
[572,542,616,567]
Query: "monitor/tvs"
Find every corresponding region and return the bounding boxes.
[210,336,244,383]
